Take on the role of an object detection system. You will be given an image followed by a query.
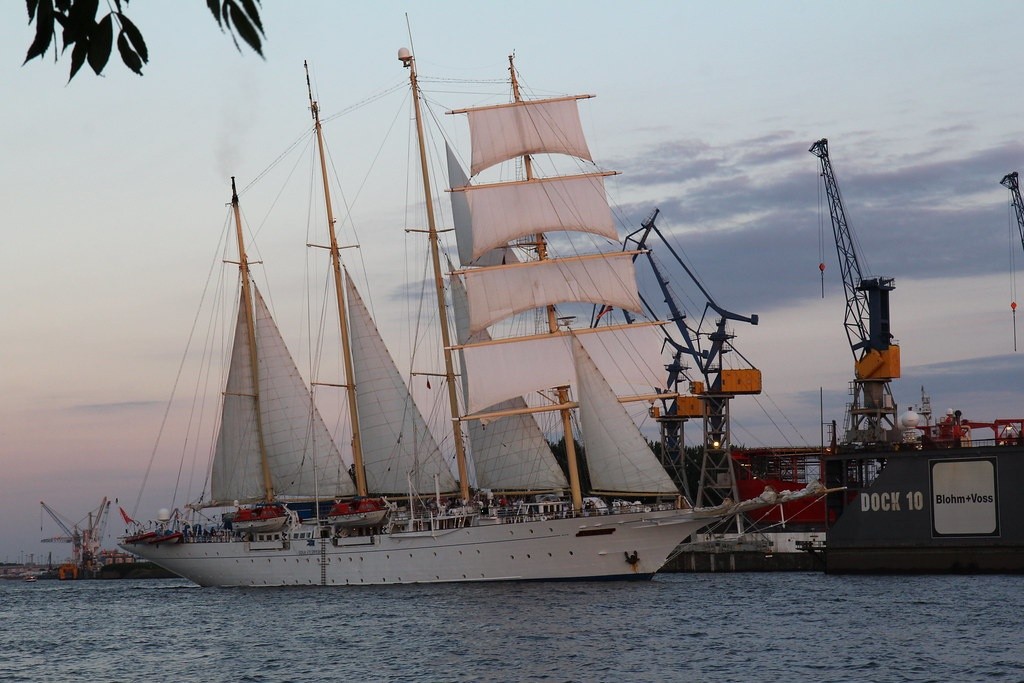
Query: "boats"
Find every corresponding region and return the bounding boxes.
[27,578,37,582]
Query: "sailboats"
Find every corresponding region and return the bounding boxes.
[116,12,848,587]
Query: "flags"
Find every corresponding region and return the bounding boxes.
[120,507,132,524]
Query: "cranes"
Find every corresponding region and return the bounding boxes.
[39,496,112,567]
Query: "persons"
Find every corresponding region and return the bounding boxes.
[455,496,466,506]
